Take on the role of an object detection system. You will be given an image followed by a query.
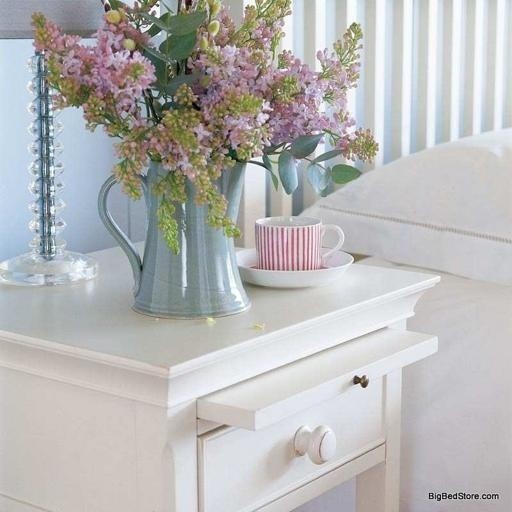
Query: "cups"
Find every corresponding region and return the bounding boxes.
[252,212,346,269]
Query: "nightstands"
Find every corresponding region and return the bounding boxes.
[1,236,443,511]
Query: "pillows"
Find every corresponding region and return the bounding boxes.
[300,122,512,286]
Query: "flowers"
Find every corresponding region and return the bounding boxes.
[28,0,379,253]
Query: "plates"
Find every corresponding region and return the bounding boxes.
[234,245,353,289]
